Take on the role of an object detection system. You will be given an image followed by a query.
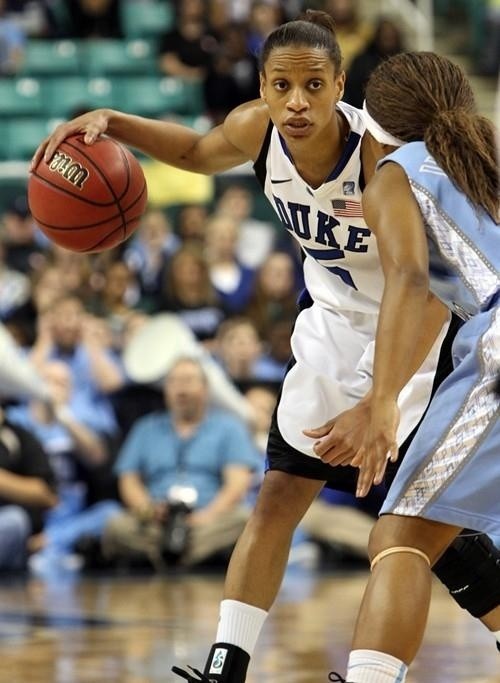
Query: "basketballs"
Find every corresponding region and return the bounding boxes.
[29,130,148,254]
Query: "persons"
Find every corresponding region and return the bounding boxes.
[27,19,500,683]
[344,50,500,683]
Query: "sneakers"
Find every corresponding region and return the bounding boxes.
[201,643,250,683]
